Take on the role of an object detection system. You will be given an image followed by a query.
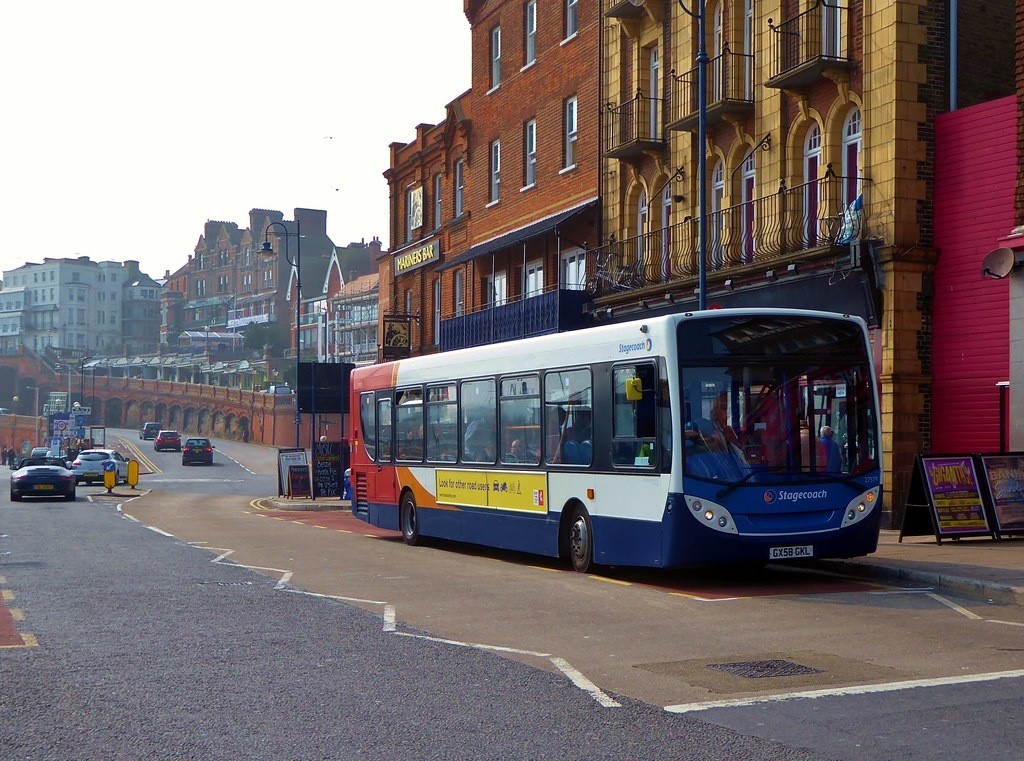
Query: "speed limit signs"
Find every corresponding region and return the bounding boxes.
[57,421,66,431]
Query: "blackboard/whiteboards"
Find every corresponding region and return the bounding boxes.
[289,465,312,497]
[313,441,343,497]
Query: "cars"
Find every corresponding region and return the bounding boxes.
[139,422,163,440]
[69,449,131,487]
[8,456,76,503]
[0,408,10,414]
[180,437,216,466]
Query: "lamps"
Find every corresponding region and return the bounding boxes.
[788,260,815,276]
[592,303,625,320]
[765,266,785,283]
[693,285,700,300]
[638,294,666,308]
[724,276,746,291]
[665,289,690,305]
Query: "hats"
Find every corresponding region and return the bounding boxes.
[799,420,809,428]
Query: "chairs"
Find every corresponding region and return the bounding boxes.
[564,439,593,465]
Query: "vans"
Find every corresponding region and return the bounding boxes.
[30,447,50,457]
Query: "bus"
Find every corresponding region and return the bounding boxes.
[349,306,885,584]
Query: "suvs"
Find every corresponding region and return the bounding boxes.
[154,429,181,452]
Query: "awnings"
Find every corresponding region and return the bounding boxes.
[433,199,598,320]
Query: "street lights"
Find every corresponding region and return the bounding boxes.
[81,356,93,405]
[257,218,302,448]
[26,386,38,447]
[205,324,210,341]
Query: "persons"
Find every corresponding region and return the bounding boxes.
[820,426,842,473]
[80,425,85,439]
[477,440,495,463]
[511,440,523,460]
[8,446,14,469]
[2,447,7,465]
[442,445,456,460]
[842,433,848,472]
[800,419,825,471]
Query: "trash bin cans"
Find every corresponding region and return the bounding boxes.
[343,475,351,500]
